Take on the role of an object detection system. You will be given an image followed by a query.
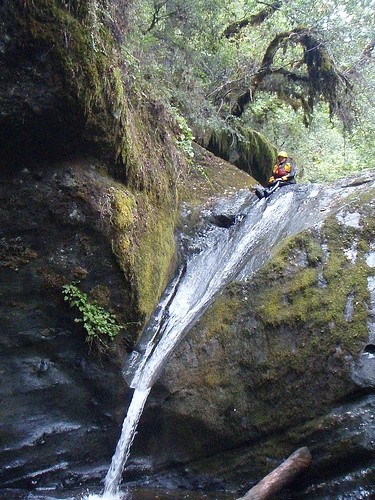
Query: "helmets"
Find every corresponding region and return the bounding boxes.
[277,151,288,158]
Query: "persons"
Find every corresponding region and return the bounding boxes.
[255,151,296,198]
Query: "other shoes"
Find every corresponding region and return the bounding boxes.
[255,189,269,198]
